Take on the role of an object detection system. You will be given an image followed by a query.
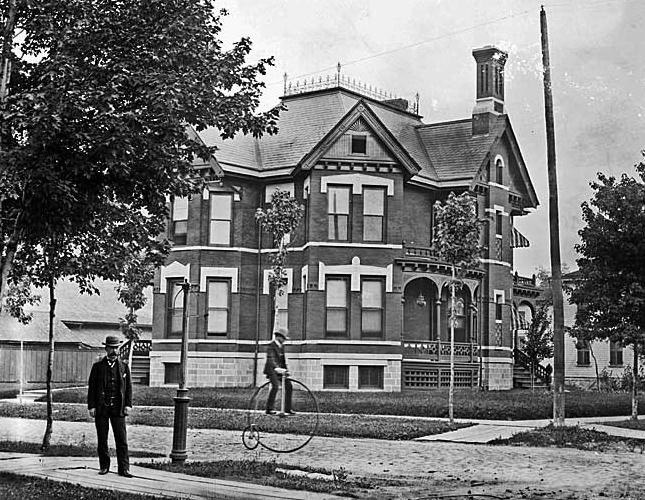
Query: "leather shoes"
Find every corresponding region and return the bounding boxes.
[285,409,295,414]
[99,468,108,474]
[118,469,133,478]
[266,409,276,414]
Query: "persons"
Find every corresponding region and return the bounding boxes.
[263,329,296,415]
[87,336,134,478]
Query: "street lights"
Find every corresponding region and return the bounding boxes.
[173,279,200,430]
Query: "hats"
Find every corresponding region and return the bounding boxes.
[274,328,289,340]
[102,337,124,347]
